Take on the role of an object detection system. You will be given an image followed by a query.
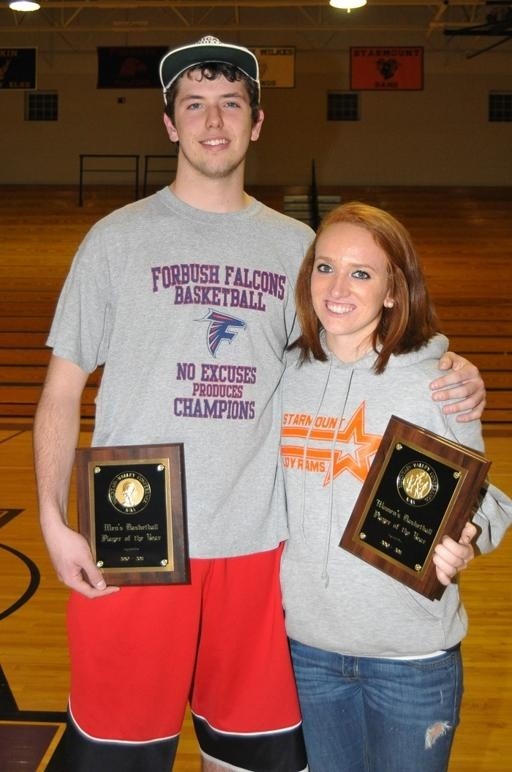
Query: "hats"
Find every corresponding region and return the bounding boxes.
[159,36,263,105]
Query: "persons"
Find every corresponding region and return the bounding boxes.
[277,199,511,771]
[30,34,486,771]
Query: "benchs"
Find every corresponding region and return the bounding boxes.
[0,183,512,422]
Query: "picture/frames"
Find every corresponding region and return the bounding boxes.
[337,414,494,600]
[247,44,424,91]
[73,443,190,585]
